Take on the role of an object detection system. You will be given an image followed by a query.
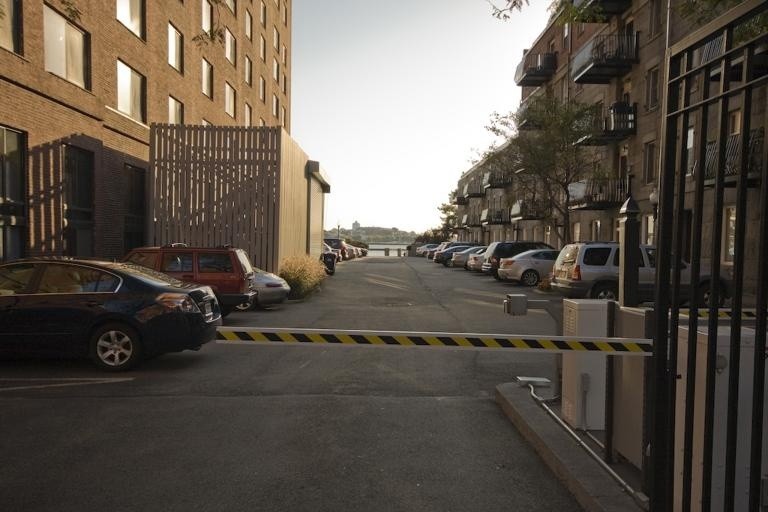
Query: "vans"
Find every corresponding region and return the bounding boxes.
[481,240,555,282]
[120,242,258,317]
[551,240,732,308]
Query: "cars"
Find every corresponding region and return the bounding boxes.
[235,265,292,314]
[322,236,368,260]
[415,242,488,272]
[498,249,559,287]
[0,256,223,375]
